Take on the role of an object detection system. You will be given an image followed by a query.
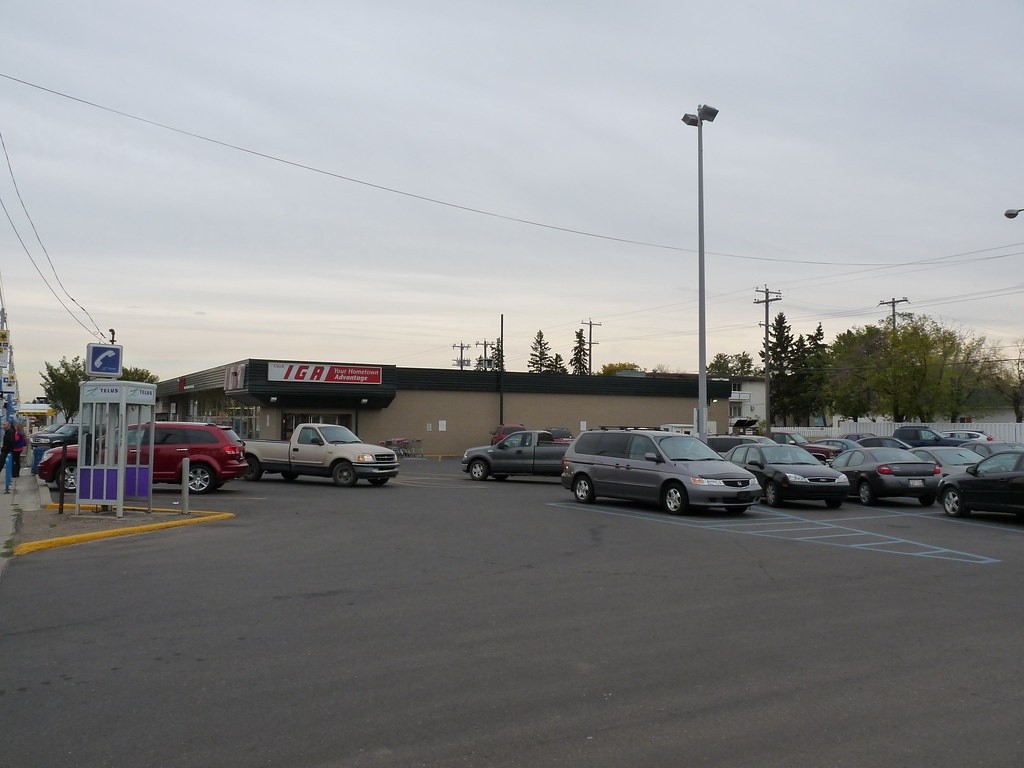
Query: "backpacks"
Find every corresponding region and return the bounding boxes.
[22,432,28,446]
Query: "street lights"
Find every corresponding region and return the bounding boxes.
[681,102,720,440]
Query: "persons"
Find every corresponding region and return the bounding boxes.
[12,422,28,477]
[0,421,15,472]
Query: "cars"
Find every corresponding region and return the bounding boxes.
[722,443,850,510]
[811,433,912,454]
[937,450,1024,518]
[33,424,64,435]
[544,429,576,441]
[907,447,993,480]
[829,446,944,507]
[958,441,1024,460]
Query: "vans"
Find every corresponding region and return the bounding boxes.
[706,435,781,459]
[561,426,763,516]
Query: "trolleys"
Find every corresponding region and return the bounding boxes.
[379,437,424,458]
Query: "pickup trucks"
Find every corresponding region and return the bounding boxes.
[461,428,573,480]
[241,423,400,487]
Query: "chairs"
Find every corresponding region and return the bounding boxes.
[777,450,788,459]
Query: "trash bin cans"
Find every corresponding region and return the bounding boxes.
[31,447,50,474]
[733,419,759,435]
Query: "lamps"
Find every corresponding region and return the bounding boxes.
[361,399,368,404]
[270,397,277,402]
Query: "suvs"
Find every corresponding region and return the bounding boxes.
[30,424,107,450]
[892,425,968,447]
[490,424,527,446]
[929,430,992,442]
[37,422,250,494]
[769,432,841,465]
